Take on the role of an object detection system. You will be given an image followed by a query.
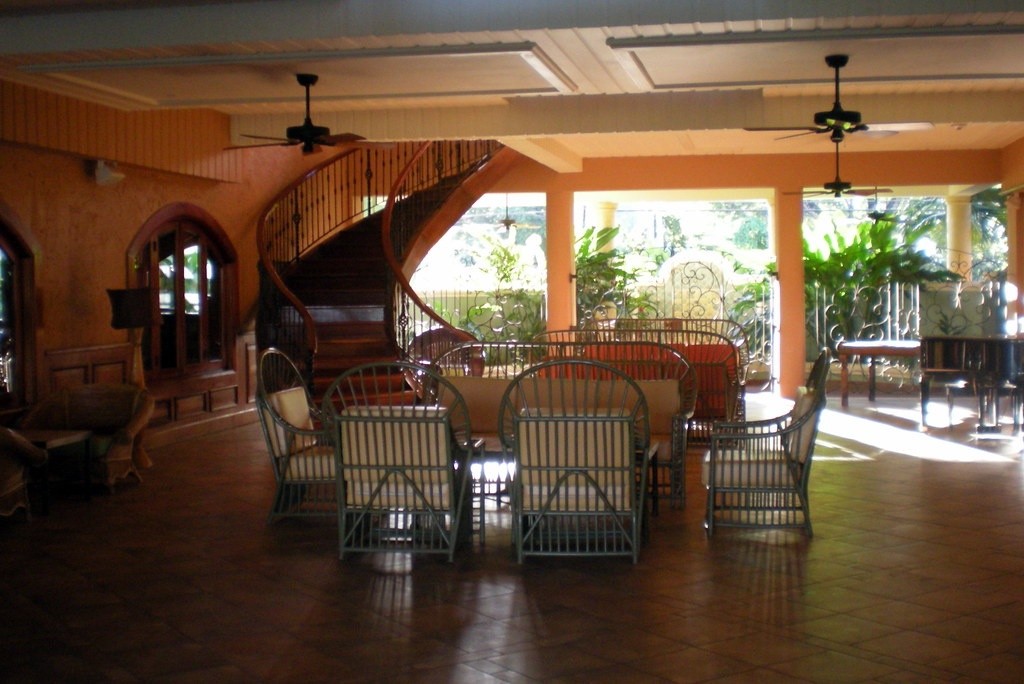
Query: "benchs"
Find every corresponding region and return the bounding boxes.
[417,342,698,509]
[920,336,1024,431]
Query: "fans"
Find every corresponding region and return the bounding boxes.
[742,55,935,141]
[223,74,392,153]
[784,142,893,198]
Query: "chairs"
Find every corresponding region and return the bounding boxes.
[496,358,661,581]
[323,363,486,569]
[701,347,830,542]
[0,427,48,530]
[252,349,334,532]
[20,382,155,494]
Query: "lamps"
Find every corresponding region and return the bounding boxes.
[834,192,841,197]
[302,143,323,156]
[829,127,844,141]
[107,286,163,387]
[94,161,124,185]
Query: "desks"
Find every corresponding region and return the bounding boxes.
[13,428,93,518]
[460,441,660,528]
[838,341,921,407]
[943,381,1020,429]
